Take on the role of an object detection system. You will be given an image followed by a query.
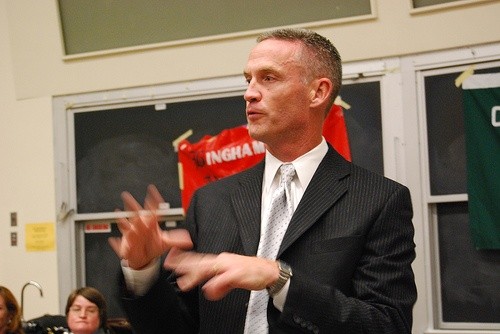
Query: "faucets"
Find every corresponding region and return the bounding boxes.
[19,280,44,328]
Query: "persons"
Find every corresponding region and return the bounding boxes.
[65,288,108,334]
[108,28,417,334]
[0,286,21,334]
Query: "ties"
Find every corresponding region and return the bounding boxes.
[243,164,296,334]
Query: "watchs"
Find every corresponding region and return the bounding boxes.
[268,260,293,297]
